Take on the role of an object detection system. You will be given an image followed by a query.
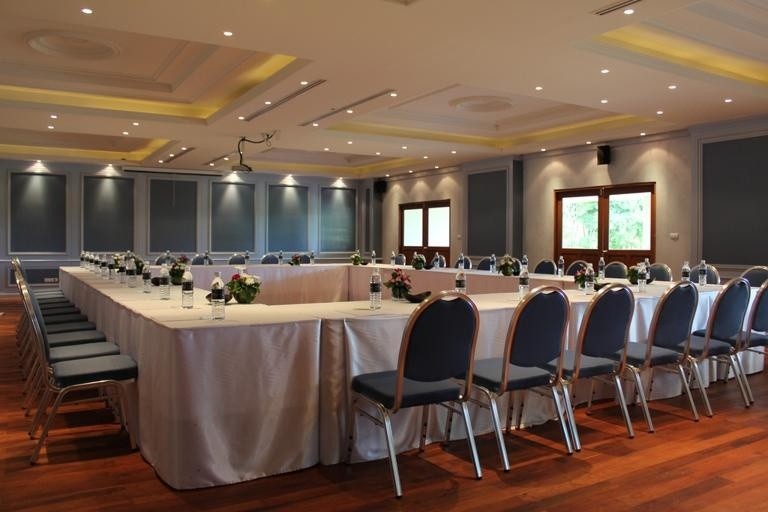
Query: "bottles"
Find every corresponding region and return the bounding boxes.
[584,263,594,295]
[489,253,496,274]
[557,255,564,277]
[680,261,690,281]
[355,250,361,256]
[456,268,467,295]
[597,257,606,281]
[458,252,465,268]
[369,267,382,309]
[521,253,529,268]
[279,249,284,264]
[244,250,250,264]
[519,265,530,298]
[309,250,315,265]
[78,249,226,322]
[371,249,440,269]
[634,257,651,295]
[697,259,707,286]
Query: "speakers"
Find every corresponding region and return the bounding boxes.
[597,145,611,164]
[373,181,386,193]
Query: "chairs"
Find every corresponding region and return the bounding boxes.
[393,254,493,270]
[154,251,311,265]
[7,255,141,466]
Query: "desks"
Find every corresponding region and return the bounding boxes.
[57,261,768,499]
